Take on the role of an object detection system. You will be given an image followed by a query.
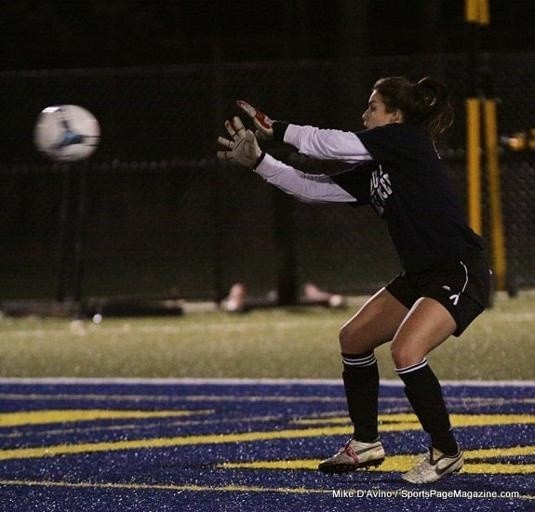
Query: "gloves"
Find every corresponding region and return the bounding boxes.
[216,114,262,171]
[236,99,278,142]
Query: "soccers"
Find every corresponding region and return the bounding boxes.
[34,105,100,162]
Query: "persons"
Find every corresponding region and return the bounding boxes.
[215,75,493,487]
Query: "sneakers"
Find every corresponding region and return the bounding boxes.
[400,441,466,486]
[318,434,387,475]
